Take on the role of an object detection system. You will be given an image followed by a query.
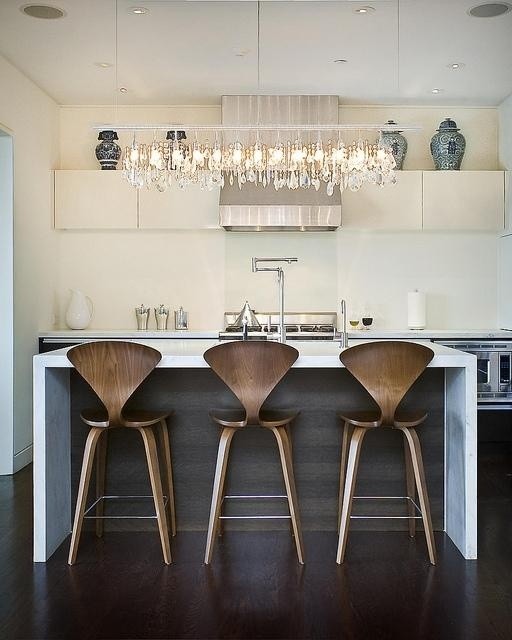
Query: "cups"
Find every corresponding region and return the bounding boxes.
[360,311,376,331]
[348,311,361,332]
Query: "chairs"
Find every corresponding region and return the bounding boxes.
[203,342,306,564]
[335,340,435,565]
[65,342,178,565]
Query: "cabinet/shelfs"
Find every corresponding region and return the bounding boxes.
[53,171,220,232]
[340,169,506,233]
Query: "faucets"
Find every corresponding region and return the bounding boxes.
[333,298,349,348]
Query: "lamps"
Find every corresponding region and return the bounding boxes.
[119,124,398,198]
